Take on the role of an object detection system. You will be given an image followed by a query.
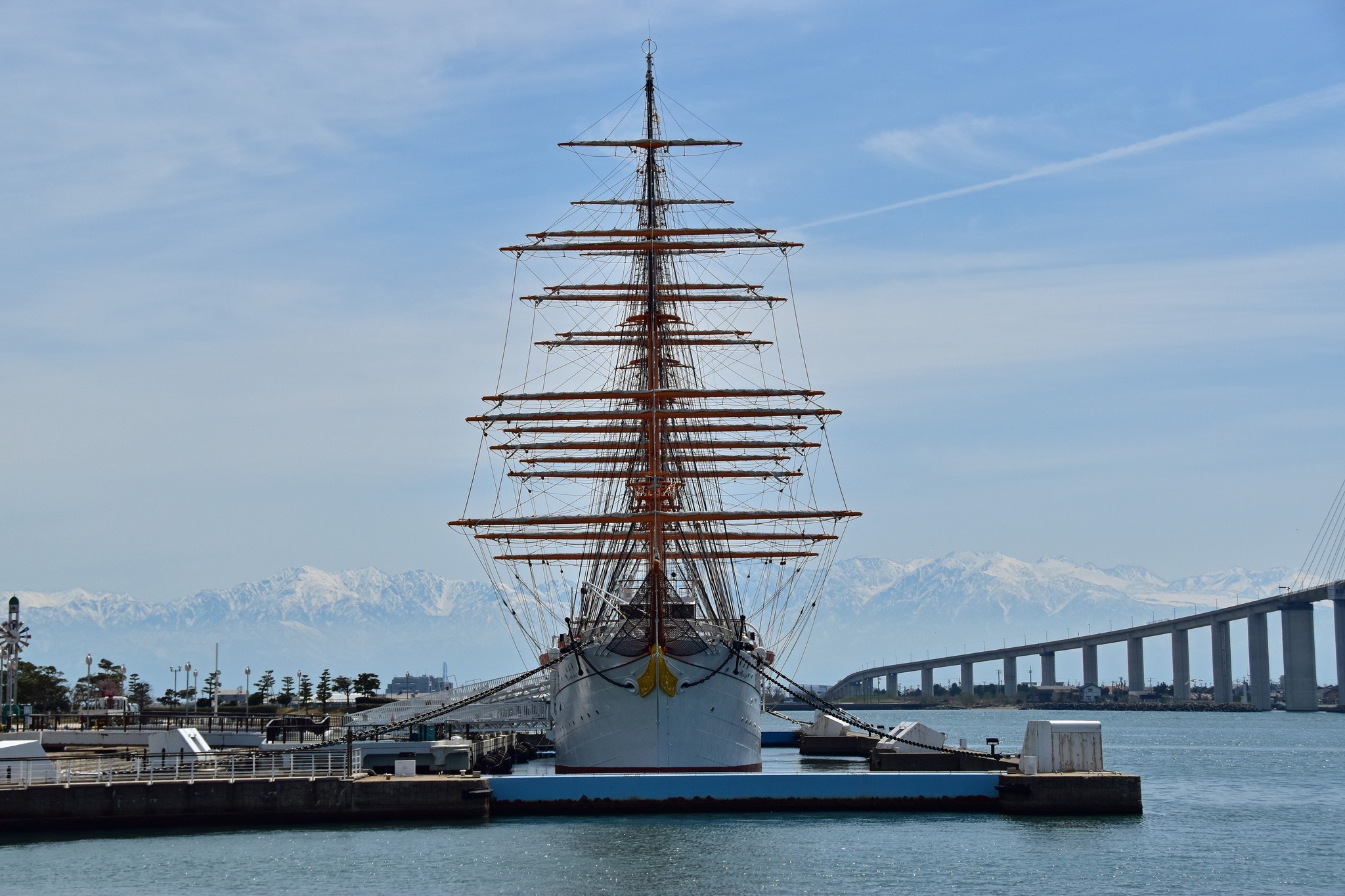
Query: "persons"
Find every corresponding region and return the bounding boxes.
[55,707,61,726]
[45,709,51,723]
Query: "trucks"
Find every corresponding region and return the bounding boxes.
[78,695,140,728]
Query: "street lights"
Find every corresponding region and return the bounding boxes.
[85,652,92,730]
[405,670,410,699]
[184,660,191,724]
[169,666,182,724]
[192,668,198,715]
[297,669,302,713]
[245,665,251,717]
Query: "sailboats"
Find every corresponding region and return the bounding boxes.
[445,21,863,775]
